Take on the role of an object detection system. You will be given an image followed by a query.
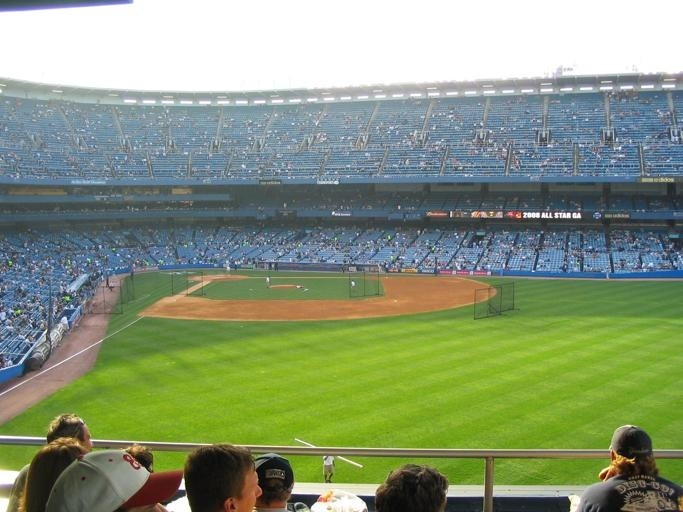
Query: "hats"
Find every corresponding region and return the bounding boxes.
[611,425,652,458]
[254,453,293,492]
[47,449,183,512]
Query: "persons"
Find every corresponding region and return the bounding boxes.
[1,88,682,367]
[5,413,449,512]
[575,424,683,512]
